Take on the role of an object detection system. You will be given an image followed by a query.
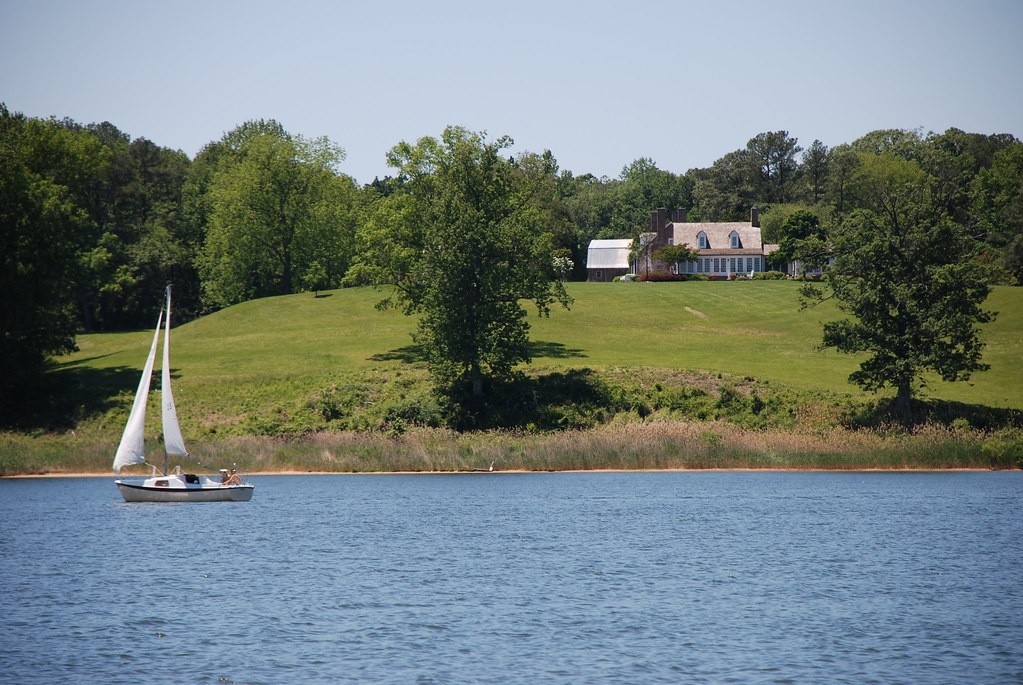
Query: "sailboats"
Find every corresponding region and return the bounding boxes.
[111,286,256,503]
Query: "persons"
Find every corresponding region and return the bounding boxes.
[221,467,241,485]
